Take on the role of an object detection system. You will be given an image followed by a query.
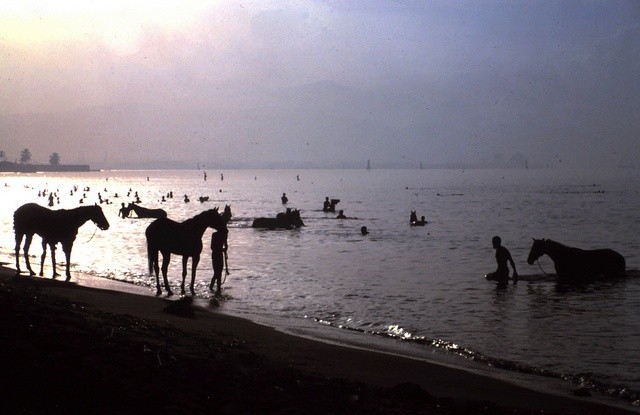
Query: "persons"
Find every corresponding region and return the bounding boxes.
[360,226,370,235]
[323,197,331,211]
[418,216,428,226]
[281,193,288,204]
[336,209,346,218]
[490,236,519,289]
[209,228,228,294]
[118,203,130,219]
[38,237,62,278]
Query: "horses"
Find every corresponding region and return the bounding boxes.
[125,202,167,220]
[527,238,625,283]
[145,207,226,295]
[251,209,302,228]
[13,203,109,279]
